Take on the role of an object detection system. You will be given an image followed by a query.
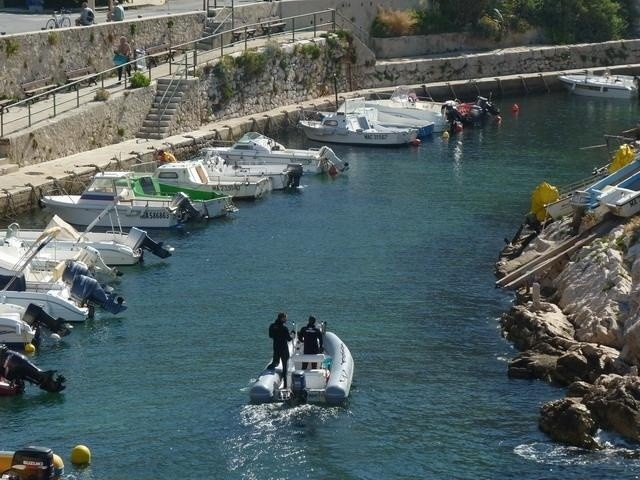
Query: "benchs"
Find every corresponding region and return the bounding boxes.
[0,14,289,115]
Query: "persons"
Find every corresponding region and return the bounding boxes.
[106,0,125,22]
[75,2,98,27]
[113,37,132,84]
[268,311,293,378]
[297,316,323,369]
[155,148,177,162]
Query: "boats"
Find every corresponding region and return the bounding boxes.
[530,143,640,222]
[297,110,422,148]
[196,130,353,179]
[306,81,505,138]
[128,171,240,221]
[556,66,640,100]
[249,329,357,405]
[0,220,128,396]
[117,158,273,201]
[183,147,305,190]
[0,444,66,480]
[0,189,175,268]
[37,169,206,230]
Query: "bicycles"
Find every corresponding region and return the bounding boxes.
[43,6,72,30]
